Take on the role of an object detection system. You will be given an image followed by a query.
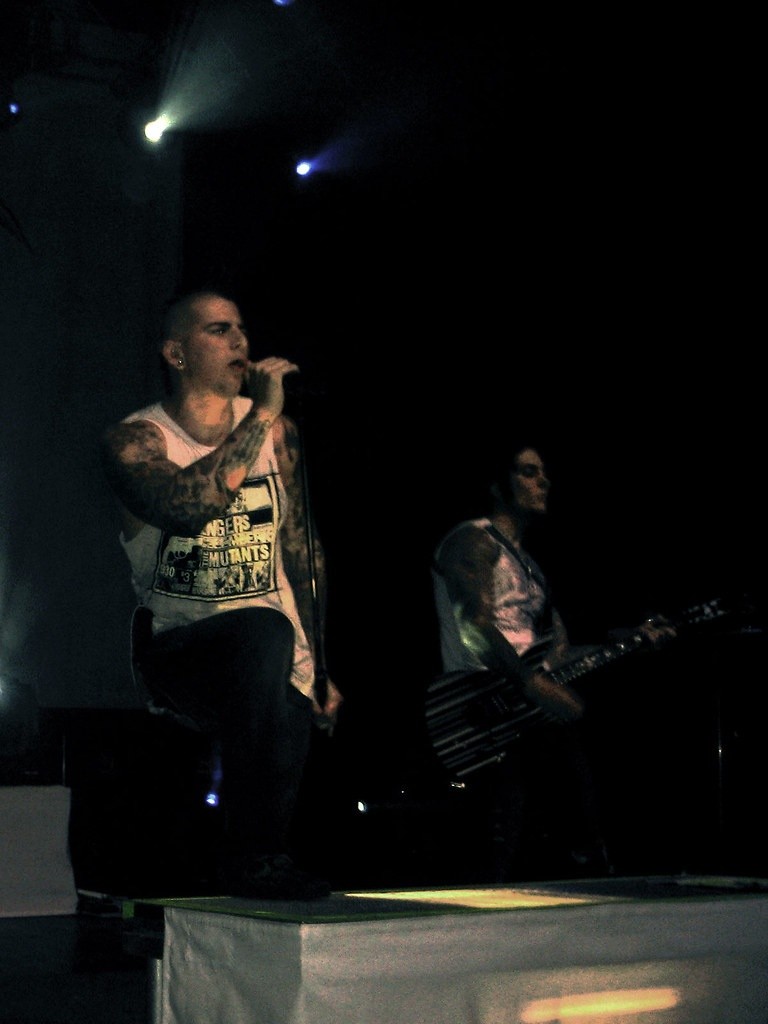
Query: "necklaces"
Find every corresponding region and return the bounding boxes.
[527,564,533,575]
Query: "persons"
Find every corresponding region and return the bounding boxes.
[106,278,341,900]
[432,435,584,880]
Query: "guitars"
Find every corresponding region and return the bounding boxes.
[415,588,756,815]
